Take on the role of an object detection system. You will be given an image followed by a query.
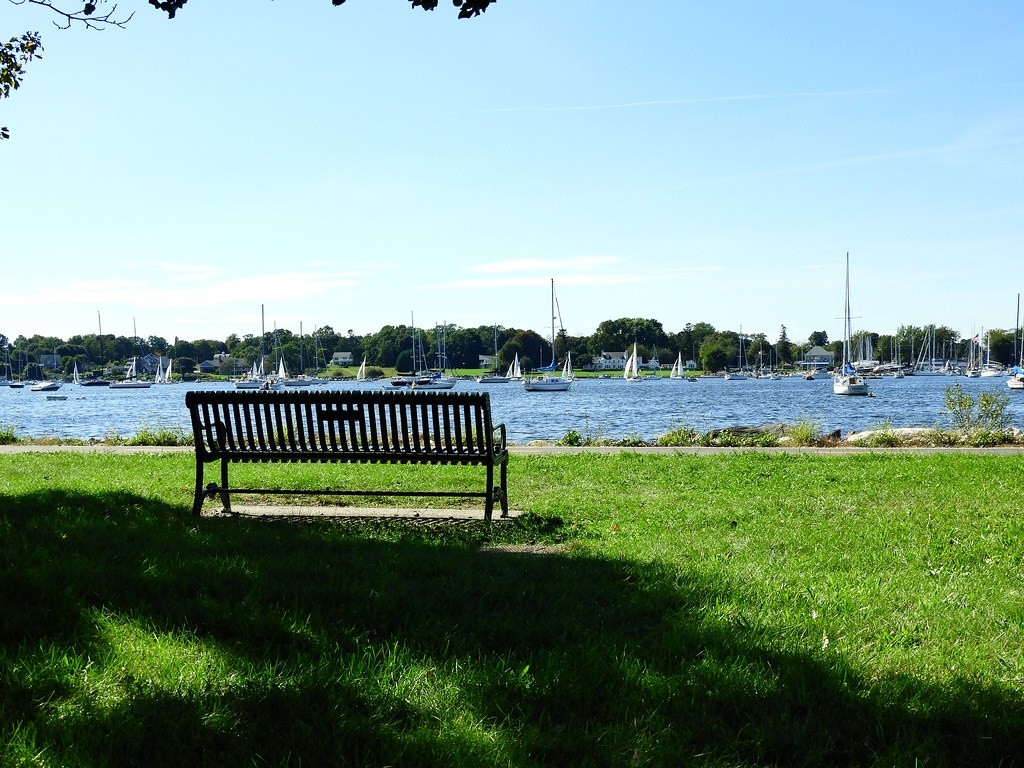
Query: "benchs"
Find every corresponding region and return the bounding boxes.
[186,390,508,527]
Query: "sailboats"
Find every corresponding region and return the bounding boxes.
[10,353,25,388]
[0,309,202,386]
[477,322,512,383]
[234,303,331,390]
[852,324,1005,380]
[1005,332,1024,389]
[518,278,575,391]
[700,324,782,380]
[562,350,580,381]
[670,351,699,380]
[356,351,368,382]
[598,337,663,381]
[506,352,522,379]
[390,310,457,389]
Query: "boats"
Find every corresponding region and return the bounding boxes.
[832,251,869,396]
[30,382,61,391]
[46,395,68,401]
[108,356,152,388]
[894,368,905,379]
[688,375,697,382]
[802,367,831,379]
